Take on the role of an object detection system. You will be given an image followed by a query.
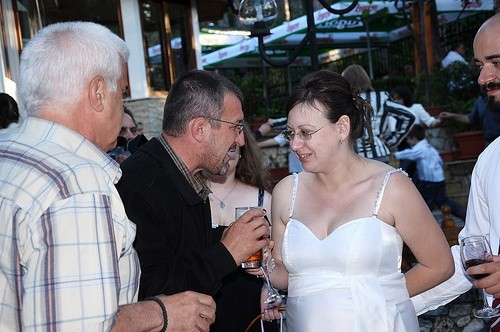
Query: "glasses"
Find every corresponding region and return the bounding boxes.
[280,124,323,142]
[206,116,247,134]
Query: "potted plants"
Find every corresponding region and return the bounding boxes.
[411,53,485,163]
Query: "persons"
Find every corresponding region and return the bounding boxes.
[266,73,456,331]
[438,41,473,100]
[0,21,218,332]
[0,93,21,128]
[204,120,281,332]
[439,84,500,150]
[246,61,469,228]
[113,68,285,332]
[403,9,500,331]
[105,105,142,166]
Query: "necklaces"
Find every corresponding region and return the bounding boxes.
[208,178,238,209]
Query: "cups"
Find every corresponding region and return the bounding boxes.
[235,207,261,269]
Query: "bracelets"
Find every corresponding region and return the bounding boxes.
[143,296,168,332]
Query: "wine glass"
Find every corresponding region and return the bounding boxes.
[460,235,500,319]
[258,246,285,303]
